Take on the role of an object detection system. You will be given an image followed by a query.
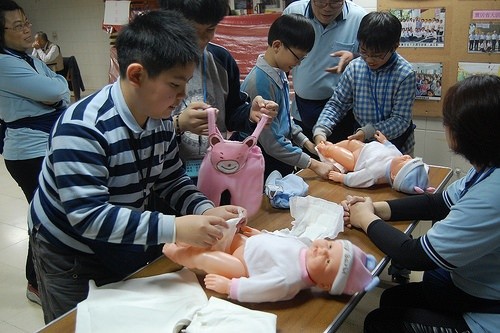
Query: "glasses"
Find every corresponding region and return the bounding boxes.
[285,45,308,62]
[4,21,33,30]
[313,1,344,9]
[358,44,392,60]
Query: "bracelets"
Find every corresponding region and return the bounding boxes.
[174,115,184,135]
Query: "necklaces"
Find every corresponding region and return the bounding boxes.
[279,72,294,138]
[126,123,156,200]
[365,60,388,124]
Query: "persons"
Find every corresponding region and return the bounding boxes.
[405,62,444,101]
[0,1,71,308]
[339,73,500,332]
[281,0,373,150]
[226,12,340,185]
[317,128,431,194]
[385,6,445,44]
[160,0,278,215]
[30,29,65,83]
[469,21,500,53]
[312,10,415,159]
[162,212,380,305]
[27,9,248,326]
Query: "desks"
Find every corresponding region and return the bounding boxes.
[35,143,456,333]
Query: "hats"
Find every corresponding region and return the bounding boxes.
[392,158,436,194]
[330,239,379,297]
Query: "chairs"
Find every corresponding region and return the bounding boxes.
[61,56,85,103]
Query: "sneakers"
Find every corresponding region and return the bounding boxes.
[25,285,43,306]
